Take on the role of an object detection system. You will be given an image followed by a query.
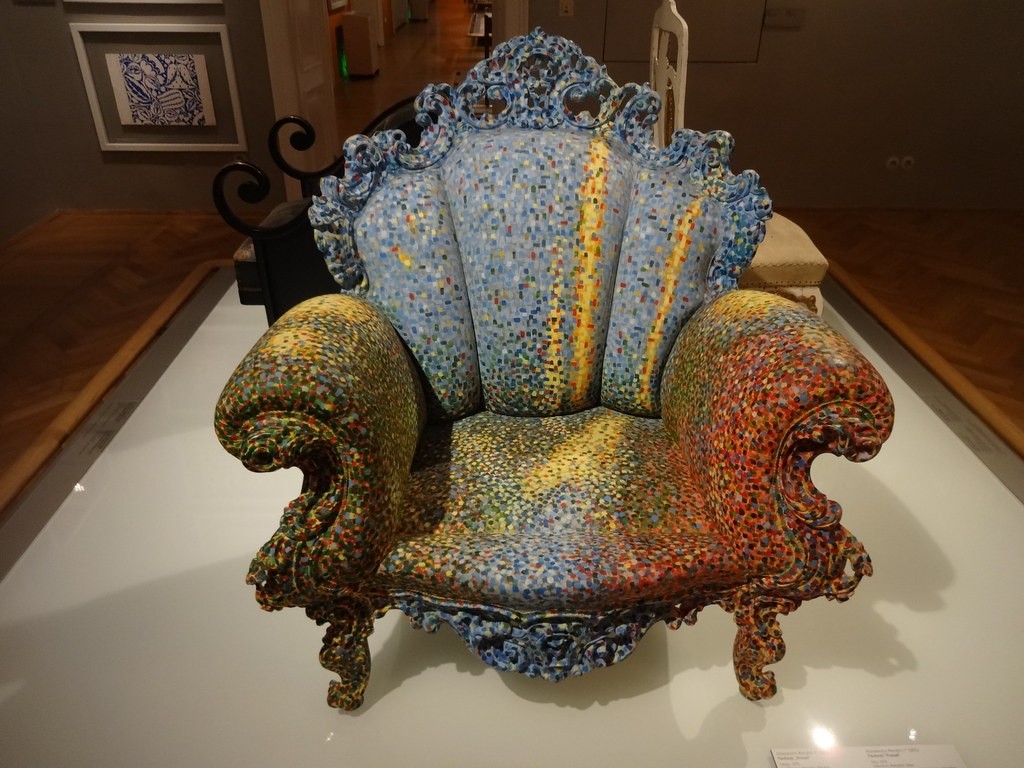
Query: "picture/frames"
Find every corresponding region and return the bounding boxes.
[68,21,248,155]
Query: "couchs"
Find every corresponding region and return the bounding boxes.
[212,26,895,711]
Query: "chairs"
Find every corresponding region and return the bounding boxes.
[648,0,830,318]
